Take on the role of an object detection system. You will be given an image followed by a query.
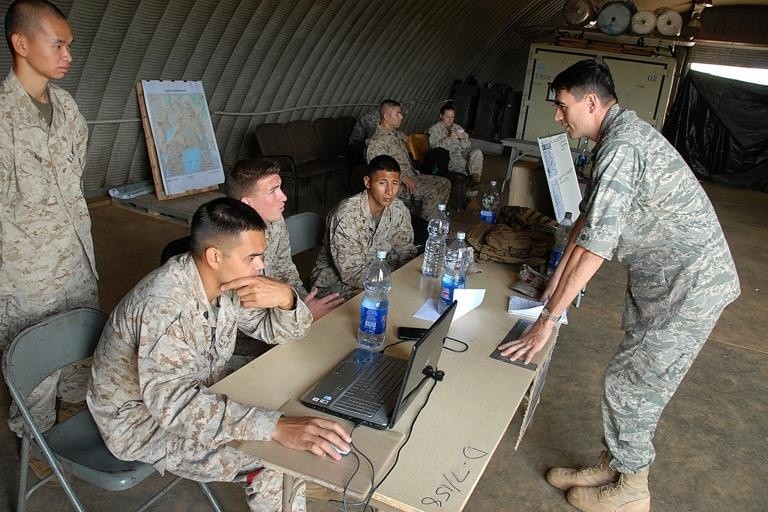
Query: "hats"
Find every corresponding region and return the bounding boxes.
[510,262,552,299]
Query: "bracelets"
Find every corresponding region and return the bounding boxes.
[539,306,562,323]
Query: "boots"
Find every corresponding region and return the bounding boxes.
[466,174,484,215]
[566,469,651,512]
[546,449,620,493]
[305,481,359,504]
[18,438,63,488]
[57,399,88,424]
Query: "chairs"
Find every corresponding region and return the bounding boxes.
[8,307,223,512]
[282,213,318,291]
[251,116,466,212]
[157,234,191,267]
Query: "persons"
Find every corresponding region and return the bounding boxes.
[305,155,417,302]
[0,1,102,488]
[79,197,351,511]
[424,101,484,183]
[224,154,347,325]
[496,58,743,512]
[366,98,451,231]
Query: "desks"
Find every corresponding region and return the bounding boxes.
[205,240,575,511]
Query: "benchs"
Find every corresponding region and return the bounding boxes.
[500,137,593,183]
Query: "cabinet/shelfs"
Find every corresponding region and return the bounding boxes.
[505,42,677,182]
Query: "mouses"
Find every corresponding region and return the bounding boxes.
[320,437,351,456]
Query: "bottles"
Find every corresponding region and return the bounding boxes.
[575,138,590,172]
[479,179,501,225]
[437,231,469,316]
[547,211,574,276]
[356,247,391,348]
[422,202,451,281]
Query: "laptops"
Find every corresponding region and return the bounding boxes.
[299,300,458,429]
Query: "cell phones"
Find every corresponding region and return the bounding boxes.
[396,326,428,341]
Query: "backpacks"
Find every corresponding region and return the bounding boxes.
[499,204,560,233]
[461,220,551,269]
